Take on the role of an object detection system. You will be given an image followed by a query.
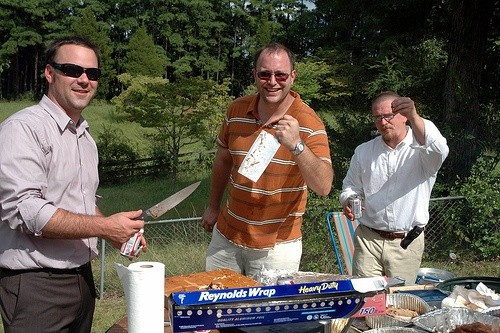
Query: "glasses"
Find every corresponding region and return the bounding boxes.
[373,112,399,122]
[256,70,291,81]
[47,61,101,80]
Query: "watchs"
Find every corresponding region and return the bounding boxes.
[289,138,305,156]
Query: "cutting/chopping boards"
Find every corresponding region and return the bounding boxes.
[164,268,265,308]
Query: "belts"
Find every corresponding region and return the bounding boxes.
[363,225,404,239]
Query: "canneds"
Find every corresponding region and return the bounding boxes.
[348,194,362,219]
[277,275,295,286]
[119,226,144,258]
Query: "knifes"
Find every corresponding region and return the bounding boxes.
[238,130,282,183]
[131,181,202,223]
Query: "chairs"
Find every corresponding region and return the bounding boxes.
[326,212,445,285]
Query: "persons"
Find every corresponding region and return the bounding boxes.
[201,44,333,278]
[0,36,146,333]
[338,91,449,284]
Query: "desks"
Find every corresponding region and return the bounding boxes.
[106,273,361,333]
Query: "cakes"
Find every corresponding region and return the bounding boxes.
[164,268,258,297]
